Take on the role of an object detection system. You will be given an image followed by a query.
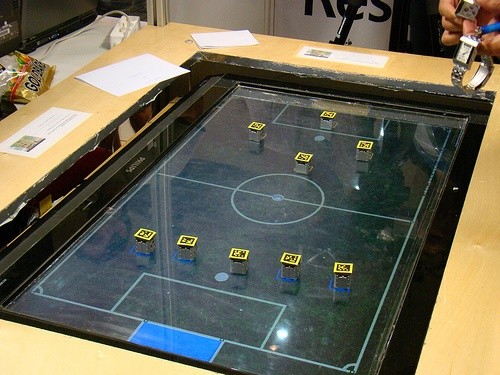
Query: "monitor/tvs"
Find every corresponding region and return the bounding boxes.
[21,0,100,54]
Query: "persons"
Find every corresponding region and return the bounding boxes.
[438,0,500,57]
[75,205,133,264]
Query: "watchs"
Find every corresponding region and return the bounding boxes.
[450,34,495,92]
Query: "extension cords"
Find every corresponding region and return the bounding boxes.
[109,14,141,50]
